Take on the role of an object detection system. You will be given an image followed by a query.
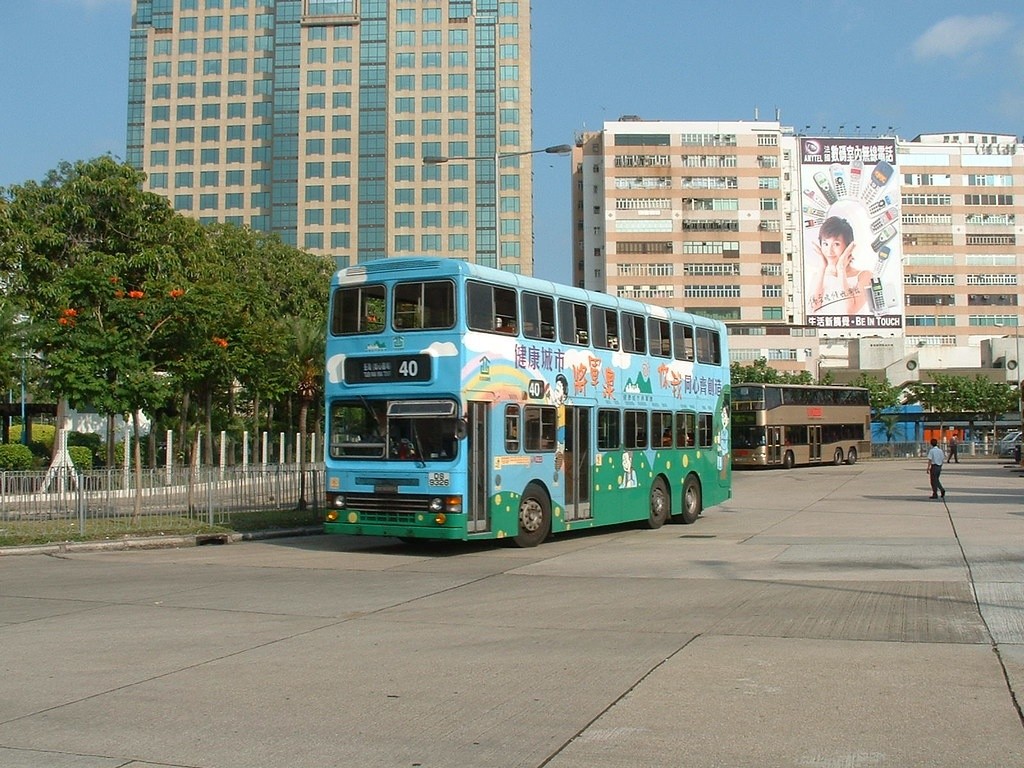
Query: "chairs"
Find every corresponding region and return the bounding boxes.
[496,326,516,334]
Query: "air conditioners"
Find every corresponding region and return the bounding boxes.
[637,132,769,274]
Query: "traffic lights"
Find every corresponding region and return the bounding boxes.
[1004,347,1020,382]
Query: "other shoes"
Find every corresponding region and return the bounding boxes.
[929,496,937,498]
[941,490,945,497]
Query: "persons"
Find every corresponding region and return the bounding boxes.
[926,439,945,499]
[810,216,872,315]
[947,433,960,463]
[663,426,674,446]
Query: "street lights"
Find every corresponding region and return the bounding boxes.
[423,143,572,271]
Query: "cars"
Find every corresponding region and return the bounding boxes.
[1000,430,1023,456]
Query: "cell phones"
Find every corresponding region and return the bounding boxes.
[872,206,900,232]
[813,172,838,204]
[805,218,826,228]
[860,160,892,206]
[871,224,897,251]
[849,160,864,203]
[804,188,830,210]
[875,247,890,275]
[828,162,847,198]
[867,196,893,217]
[802,206,826,217]
[870,278,885,311]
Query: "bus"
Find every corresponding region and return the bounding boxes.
[324,255,734,548]
[870,405,927,443]
[731,384,872,469]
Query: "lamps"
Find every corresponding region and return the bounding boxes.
[803,125,811,134]
[854,125,861,132]
[869,125,877,134]
[839,125,845,132]
[821,126,827,133]
[885,126,894,135]
